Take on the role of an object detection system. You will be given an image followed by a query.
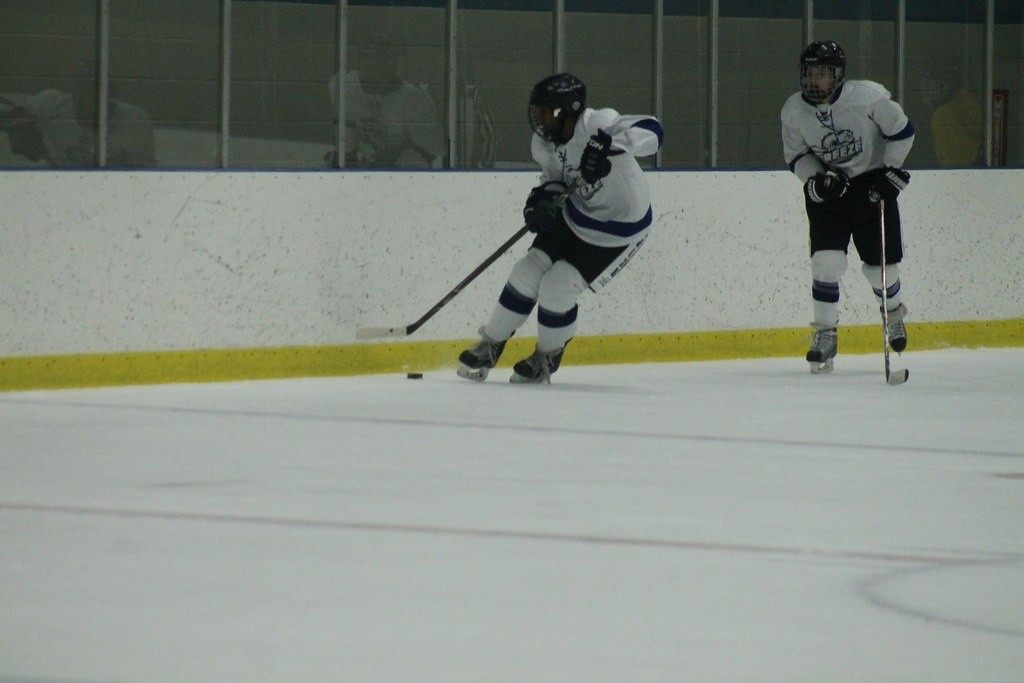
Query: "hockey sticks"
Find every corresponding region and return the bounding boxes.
[355,175,587,338]
[879,199,910,385]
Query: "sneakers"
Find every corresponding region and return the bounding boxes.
[509,337,574,384]
[806,322,838,374]
[880,303,907,356]
[457,330,516,381]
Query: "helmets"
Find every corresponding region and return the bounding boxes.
[527,72,587,147]
[798,40,848,102]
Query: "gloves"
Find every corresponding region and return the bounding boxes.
[580,128,612,185]
[523,181,568,233]
[808,168,850,203]
[863,166,911,209]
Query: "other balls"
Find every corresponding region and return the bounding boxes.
[407,372,424,379]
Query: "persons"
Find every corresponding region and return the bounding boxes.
[781,41,916,363]
[458,72,665,382]
[6,65,157,169]
[328,35,443,167]
